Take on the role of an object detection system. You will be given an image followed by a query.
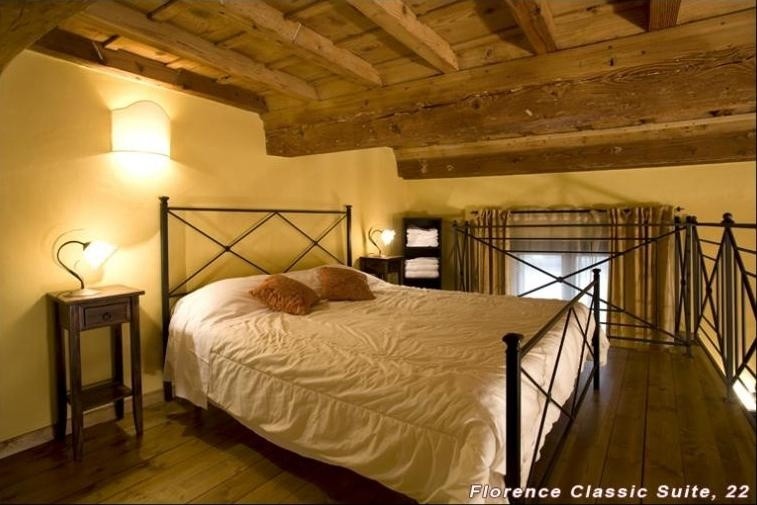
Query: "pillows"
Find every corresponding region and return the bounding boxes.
[247,274,320,315]
[319,267,376,300]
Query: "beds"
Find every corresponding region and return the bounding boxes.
[158,193,610,505]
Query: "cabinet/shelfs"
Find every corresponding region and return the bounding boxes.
[402,216,443,290]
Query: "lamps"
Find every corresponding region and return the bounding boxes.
[56,238,116,297]
[110,98,173,163]
[367,226,397,258]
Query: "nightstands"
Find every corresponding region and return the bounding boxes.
[359,256,404,286]
[44,282,146,462]
